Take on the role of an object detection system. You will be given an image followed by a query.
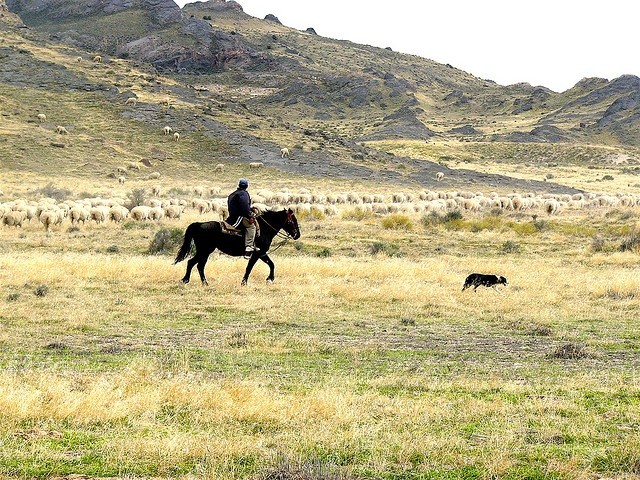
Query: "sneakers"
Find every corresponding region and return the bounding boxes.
[245,246,260,252]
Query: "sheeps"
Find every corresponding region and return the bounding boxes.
[124,97,137,107]
[93,55,102,63]
[436,171,444,182]
[192,185,204,198]
[55,125,67,134]
[209,185,222,198]
[76,57,83,62]
[127,161,140,170]
[173,132,181,142]
[249,162,264,169]
[163,126,173,136]
[149,171,161,181]
[36,113,48,124]
[280,148,291,158]
[213,163,225,174]
[1,186,640,231]
[117,176,127,184]
[116,166,128,175]
[150,184,164,197]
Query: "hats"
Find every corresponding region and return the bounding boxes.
[239,180,248,185]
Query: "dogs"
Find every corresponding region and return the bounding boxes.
[461,272,509,294]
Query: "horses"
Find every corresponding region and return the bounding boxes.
[174,208,302,289]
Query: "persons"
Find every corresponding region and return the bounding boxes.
[226,177,262,256]
